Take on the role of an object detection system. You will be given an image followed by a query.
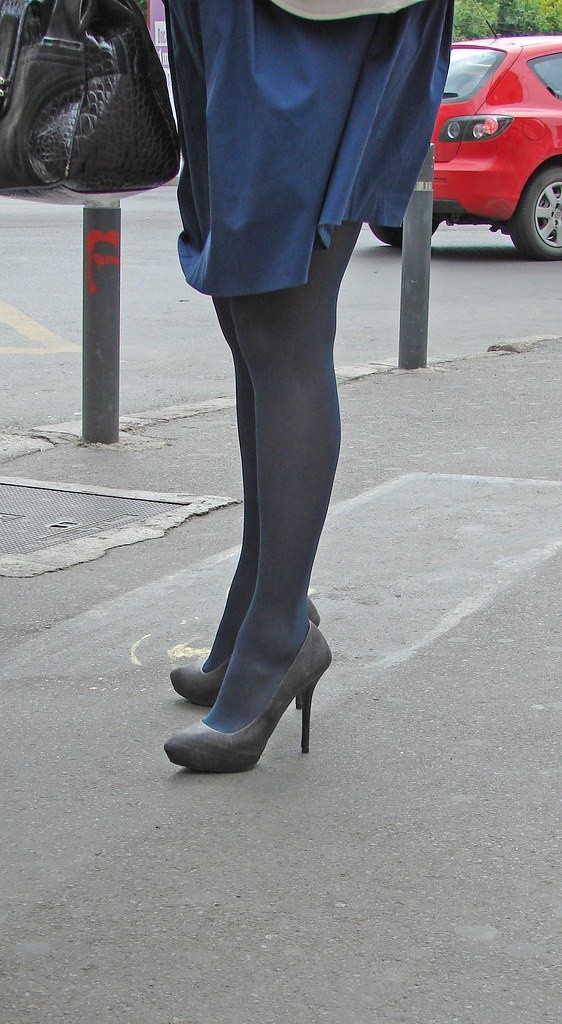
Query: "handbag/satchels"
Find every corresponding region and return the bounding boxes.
[0,0,180,205]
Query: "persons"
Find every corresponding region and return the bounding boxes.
[161,0,454,770]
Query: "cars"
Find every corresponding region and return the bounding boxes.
[368,0,562,261]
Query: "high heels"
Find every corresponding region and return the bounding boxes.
[164,619,332,772]
[170,596,321,707]
